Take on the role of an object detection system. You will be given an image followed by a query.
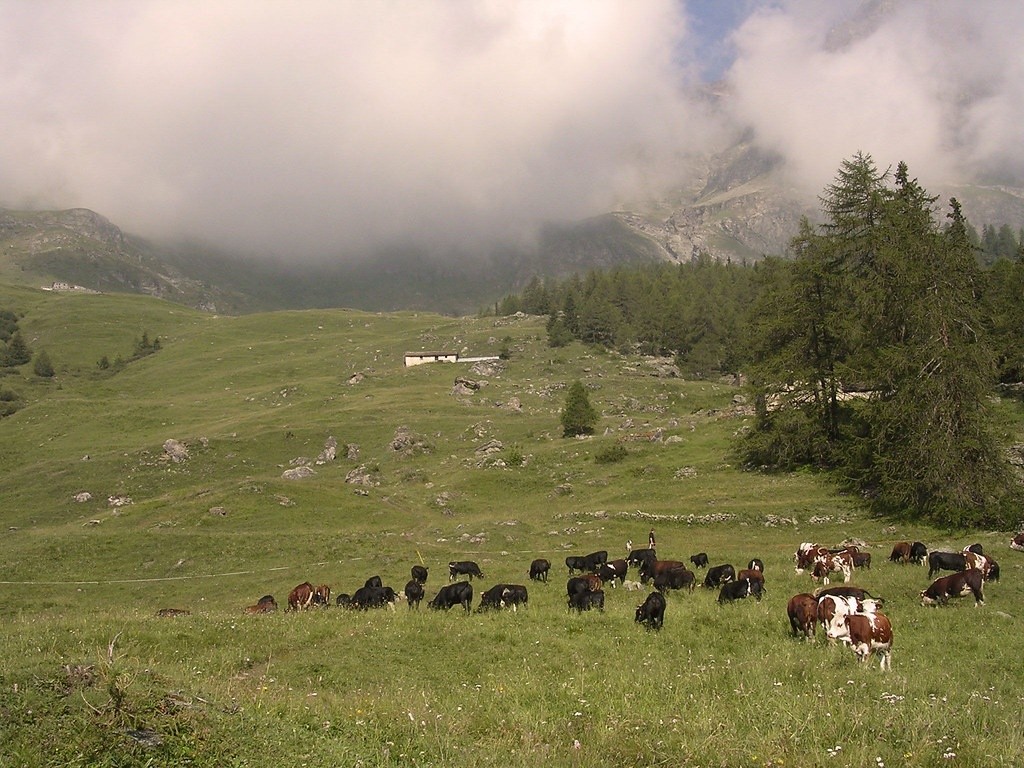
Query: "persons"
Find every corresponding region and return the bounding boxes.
[649,528,656,548]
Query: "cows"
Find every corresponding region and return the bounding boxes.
[792,541,872,585]
[1009,533,1024,552]
[244,582,330,614]
[634,592,667,631]
[889,541,928,567]
[427,581,473,616]
[404,565,429,611]
[927,543,1000,584]
[787,587,892,672]
[336,575,401,612]
[688,552,709,569]
[920,569,985,607]
[448,561,484,583]
[527,559,551,582]
[625,548,696,595]
[565,551,628,613]
[699,559,767,605]
[477,584,528,612]
